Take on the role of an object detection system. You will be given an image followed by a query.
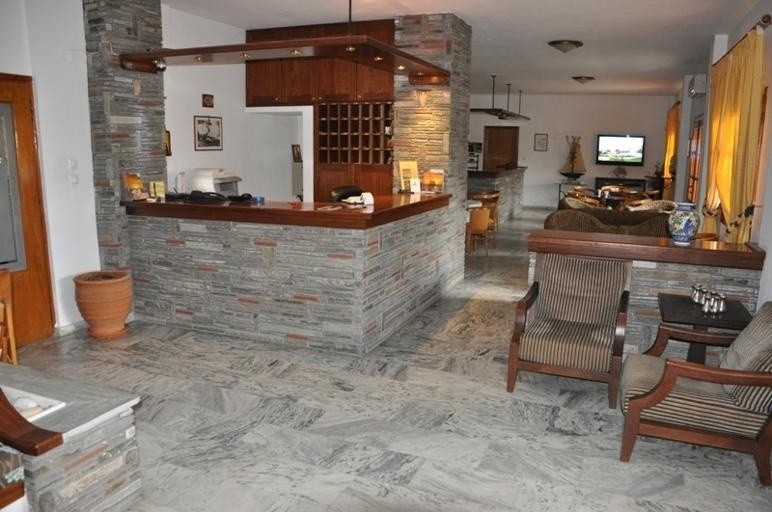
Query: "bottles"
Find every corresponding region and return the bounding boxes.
[691,284,727,314]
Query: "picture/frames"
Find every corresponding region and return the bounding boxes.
[292,145,302,162]
[534,133,548,151]
[194,116,223,151]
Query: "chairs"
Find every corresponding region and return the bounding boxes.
[466,203,501,258]
[544,184,678,239]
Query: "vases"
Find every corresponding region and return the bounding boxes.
[667,203,700,246]
[73,269,133,340]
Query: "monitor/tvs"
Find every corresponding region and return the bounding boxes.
[595,134,645,166]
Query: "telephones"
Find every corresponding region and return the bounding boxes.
[189,190,226,202]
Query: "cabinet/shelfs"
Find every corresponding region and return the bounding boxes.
[357,63,394,102]
[247,60,282,107]
[314,102,394,201]
[283,59,355,106]
